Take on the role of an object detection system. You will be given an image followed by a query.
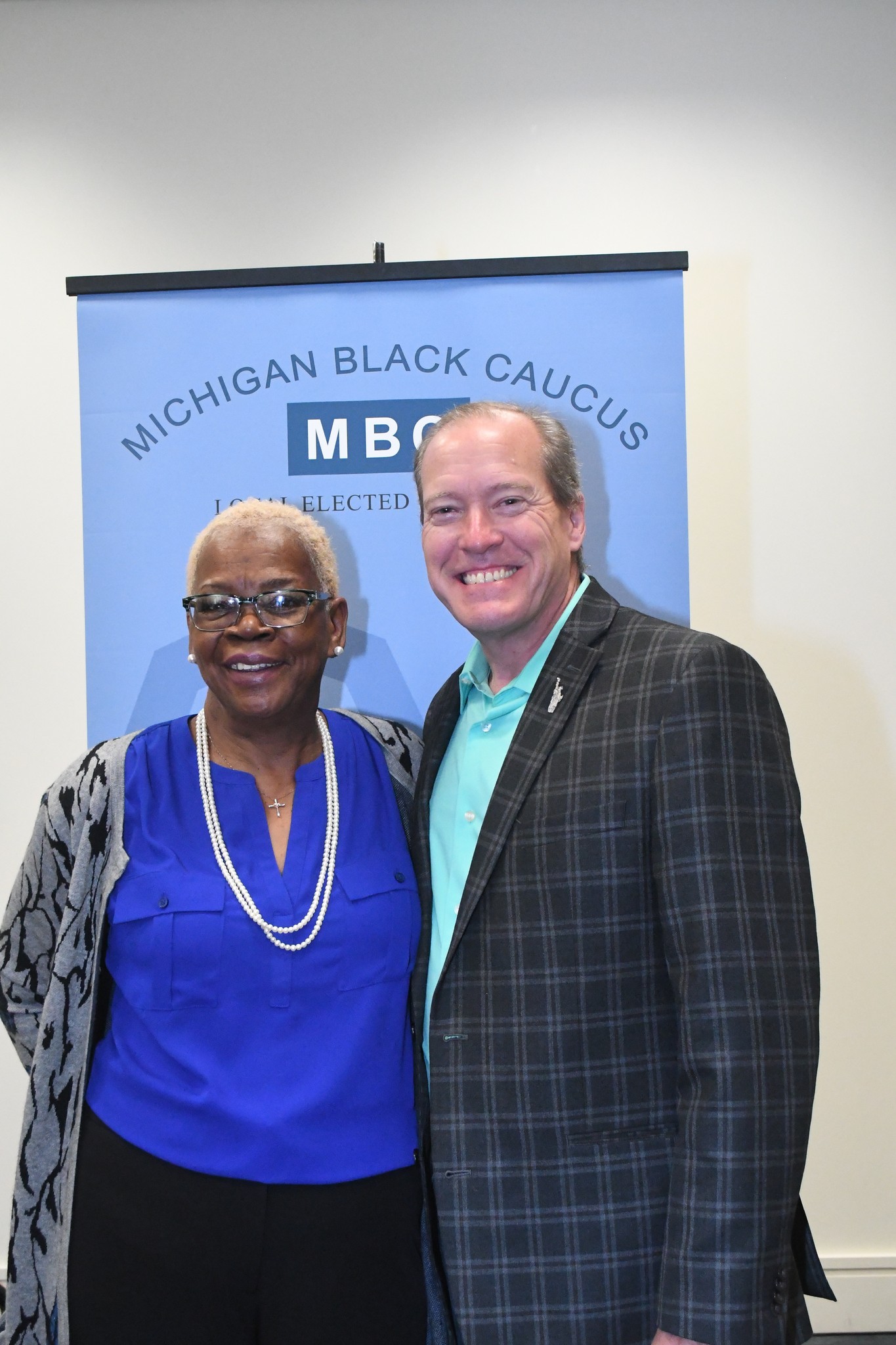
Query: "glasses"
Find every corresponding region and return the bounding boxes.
[182,588,335,631]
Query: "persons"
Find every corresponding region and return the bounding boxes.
[413,400,837,1345]
[2,496,426,1344]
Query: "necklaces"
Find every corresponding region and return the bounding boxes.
[195,708,340,952]
